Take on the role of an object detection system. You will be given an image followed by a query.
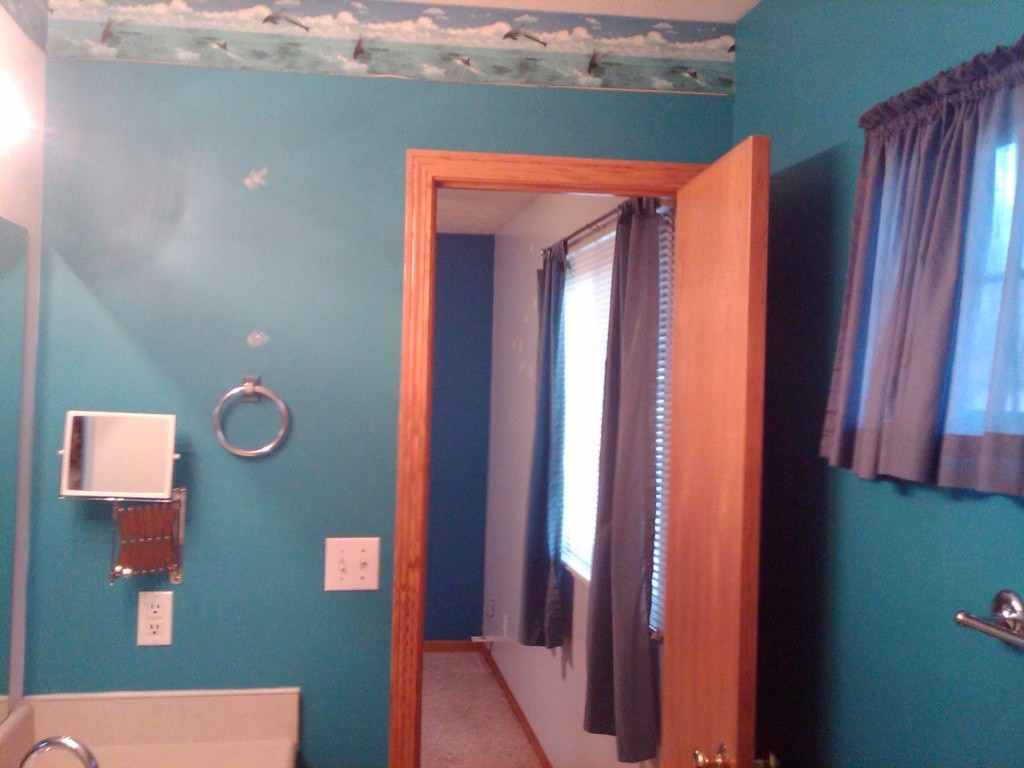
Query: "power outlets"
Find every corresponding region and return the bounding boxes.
[136,589,175,647]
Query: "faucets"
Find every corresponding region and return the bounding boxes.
[18,735,103,767]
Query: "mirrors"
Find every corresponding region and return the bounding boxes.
[0,214,30,725]
[59,410,179,501]
